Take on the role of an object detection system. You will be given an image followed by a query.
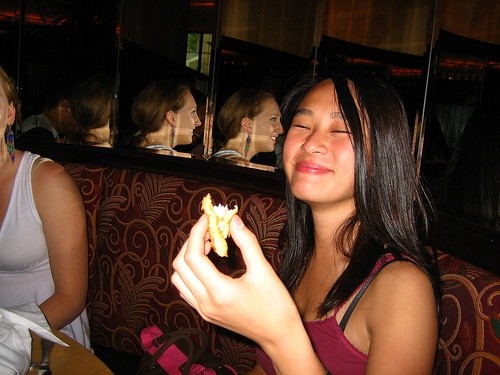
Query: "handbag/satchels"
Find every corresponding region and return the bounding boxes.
[137,325,238,375]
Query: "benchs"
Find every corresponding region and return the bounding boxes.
[15,140,500,375]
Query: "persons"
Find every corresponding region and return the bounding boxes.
[20,96,82,143]
[131,77,201,158]
[0,68,89,353]
[171,61,439,375]
[213,88,283,163]
[69,84,117,149]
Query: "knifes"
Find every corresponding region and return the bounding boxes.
[37,338,54,375]
